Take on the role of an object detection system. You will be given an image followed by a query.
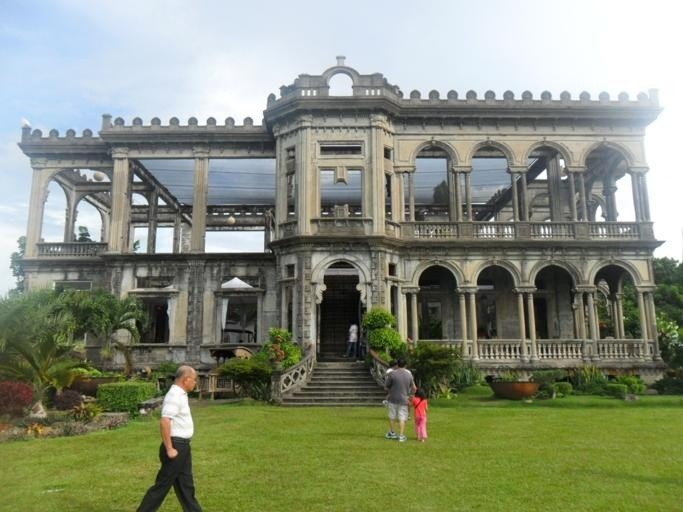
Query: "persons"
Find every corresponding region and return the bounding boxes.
[132,365,203,512]
[342,321,359,358]
[382,358,428,443]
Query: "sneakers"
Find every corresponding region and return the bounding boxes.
[398,435,408,443]
[385,431,398,439]
[381,400,388,408]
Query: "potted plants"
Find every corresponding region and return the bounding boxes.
[487,367,543,400]
[261,326,292,373]
